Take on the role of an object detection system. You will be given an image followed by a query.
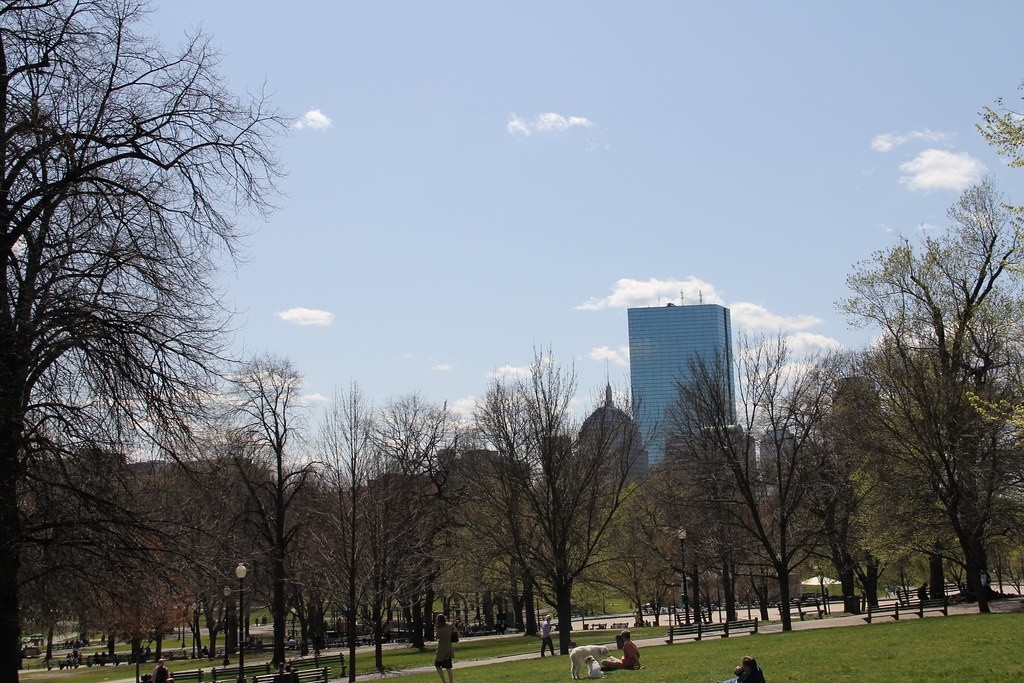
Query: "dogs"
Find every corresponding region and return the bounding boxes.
[570,645,610,679]
[584,655,604,678]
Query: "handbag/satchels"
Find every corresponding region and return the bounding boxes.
[451,625,459,642]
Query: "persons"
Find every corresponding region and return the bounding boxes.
[262,615,267,624]
[634,612,641,626]
[202,645,209,655]
[885,587,890,598]
[67,649,83,668]
[151,658,169,683]
[435,614,454,683]
[718,655,766,683]
[917,583,929,600]
[140,645,151,658]
[602,629,642,669]
[94,651,121,666]
[496,620,507,635]
[540,615,555,657]
[287,634,296,648]
[255,617,258,626]
[65,638,90,649]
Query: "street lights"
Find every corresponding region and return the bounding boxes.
[236,562,247,683]
[677,526,693,624]
[222,585,230,665]
[290,608,296,638]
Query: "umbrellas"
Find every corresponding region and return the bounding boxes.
[802,575,842,589]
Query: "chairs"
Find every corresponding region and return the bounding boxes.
[777,599,825,623]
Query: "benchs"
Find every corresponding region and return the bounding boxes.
[590,623,607,630]
[190,648,223,660]
[676,610,713,627]
[58,660,77,670]
[249,642,296,653]
[142,650,186,662]
[142,668,204,683]
[466,626,496,637]
[251,667,331,683]
[863,597,949,624]
[57,642,76,649]
[87,654,131,667]
[287,652,346,681]
[613,622,629,629]
[212,663,272,683]
[895,583,966,605]
[665,617,759,645]
